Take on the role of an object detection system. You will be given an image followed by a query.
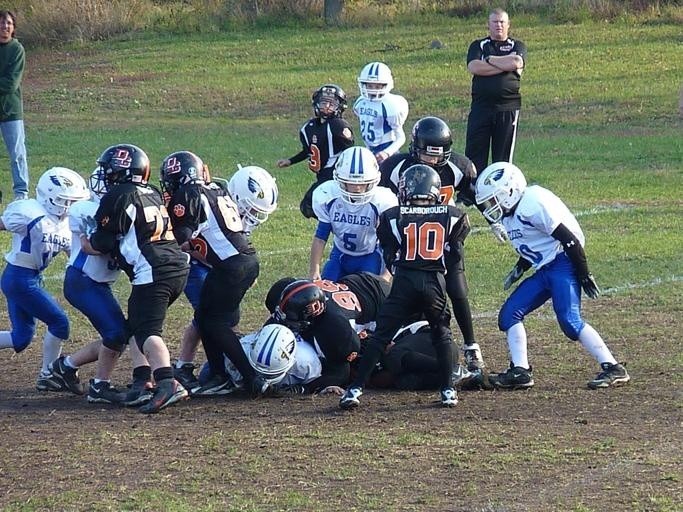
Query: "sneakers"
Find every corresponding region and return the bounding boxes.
[440,385,459,408]
[339,386,363,408]
[119,380,155,406]
[449,372,484,392]
[138,376,189,414]
[47,353,84,396]
[170,358,198,388]
[35,368,69,392]
[461,341,486,372]
[587,361,631,389]
[452,363,487,391]
[265,376,305,398]
[248,375,271,402]
[190,374,236,396]
[487,361,535,389]
[87,378,128,405]
[12,195,28,201]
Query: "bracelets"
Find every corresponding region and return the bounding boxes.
[485,53,491,64]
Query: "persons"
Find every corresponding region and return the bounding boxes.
[0,10,29,200]
[456,6,528,207]
[352,60,410,166]
[475,161,630,390]
[277,84,355,221]
[48,115,508,414]
[0,166,90,392]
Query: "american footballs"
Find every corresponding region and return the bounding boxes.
[376,368,392,387]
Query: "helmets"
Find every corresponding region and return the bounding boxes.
[408,116,453,169]
[249,323,297,385]
[158,151,207,205]
[474,161,527,224]
[357,61,395,102]
[274,279,326,331]
[332,145,382,207]
[312,83,348,120]
[89,165,108,202]
[227,165,279,231]
[90,144,151,197]
[397,163,442,206]
[36,166,91,216]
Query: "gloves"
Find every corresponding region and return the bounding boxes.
[489,222,509,245]
[504,267,524,291]
[580,271,600,301]
